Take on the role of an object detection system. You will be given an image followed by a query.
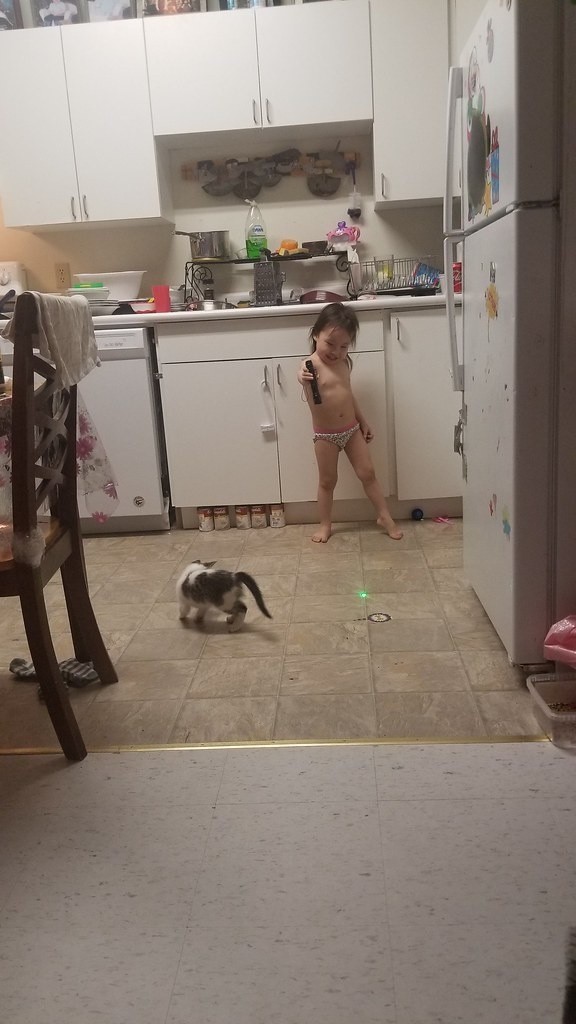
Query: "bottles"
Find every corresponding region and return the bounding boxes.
[203,278,214,300]
[347,193,360,218]
[245,198,267,258]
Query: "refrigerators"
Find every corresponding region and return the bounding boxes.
[444,0,576,676]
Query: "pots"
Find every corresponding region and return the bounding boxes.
[169,287,193,303]
[172,231,230,259]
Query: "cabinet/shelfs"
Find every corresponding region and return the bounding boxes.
[0,0,488,230]
[153,305,463,529]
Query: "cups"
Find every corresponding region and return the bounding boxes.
[152,286,170,312]
[374,255,394,282]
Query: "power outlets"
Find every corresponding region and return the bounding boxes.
[55,263,70,289]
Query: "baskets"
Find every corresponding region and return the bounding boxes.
[347,254,440,296]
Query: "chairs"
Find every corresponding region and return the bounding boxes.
[0,291,119,760]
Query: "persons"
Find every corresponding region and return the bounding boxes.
[297,302,403,542]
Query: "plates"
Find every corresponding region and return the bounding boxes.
[87,299,120,316]
[169,303,189,312]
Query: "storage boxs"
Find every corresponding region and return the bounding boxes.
[526,672,576,749]
[73,270,147,300]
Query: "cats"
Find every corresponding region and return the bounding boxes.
[175,559,273,634]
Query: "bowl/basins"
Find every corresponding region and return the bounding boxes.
[72,271,149,300]
[128,302,156,313]
[302,241,328,256]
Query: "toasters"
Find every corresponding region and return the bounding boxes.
[0,261,29,296]
